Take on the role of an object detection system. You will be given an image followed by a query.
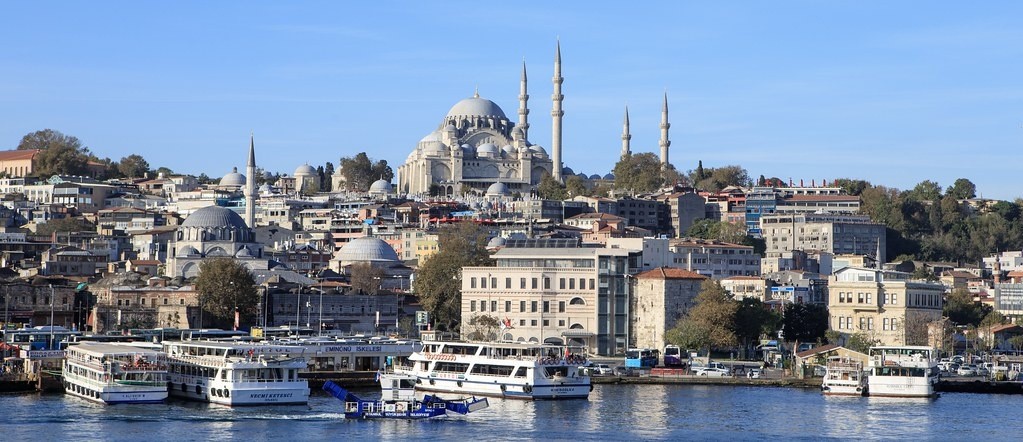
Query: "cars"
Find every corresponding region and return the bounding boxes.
[747,368,761,379]
[596,363,613,376]
[696,369,727,379]
[0,315,433,355]
[935,350,995,381]
[627,366,640,377]
[585,362,598,374]
[613,367,629,378]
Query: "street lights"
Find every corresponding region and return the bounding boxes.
[537,271,546,354]
[46,283,55,353]
[486,270,498,344]
[229,281,239,345]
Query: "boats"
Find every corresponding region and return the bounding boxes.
[319,378,491,420]
[818,344,940,400]
[61,343,169,404]
[373,318,598,405]
[157,333,312,407]
[20,347,64,392]
[0,357,39,393]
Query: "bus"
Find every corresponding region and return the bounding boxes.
[623,349,662,369]
[663,342,691,367]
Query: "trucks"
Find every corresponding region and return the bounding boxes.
[691,362,732,375]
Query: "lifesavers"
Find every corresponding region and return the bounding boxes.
[537,357,586,365]
[426,353,456,361]
[396,403,403,412]
[47,361,53,367]
[121,363,168,371]
